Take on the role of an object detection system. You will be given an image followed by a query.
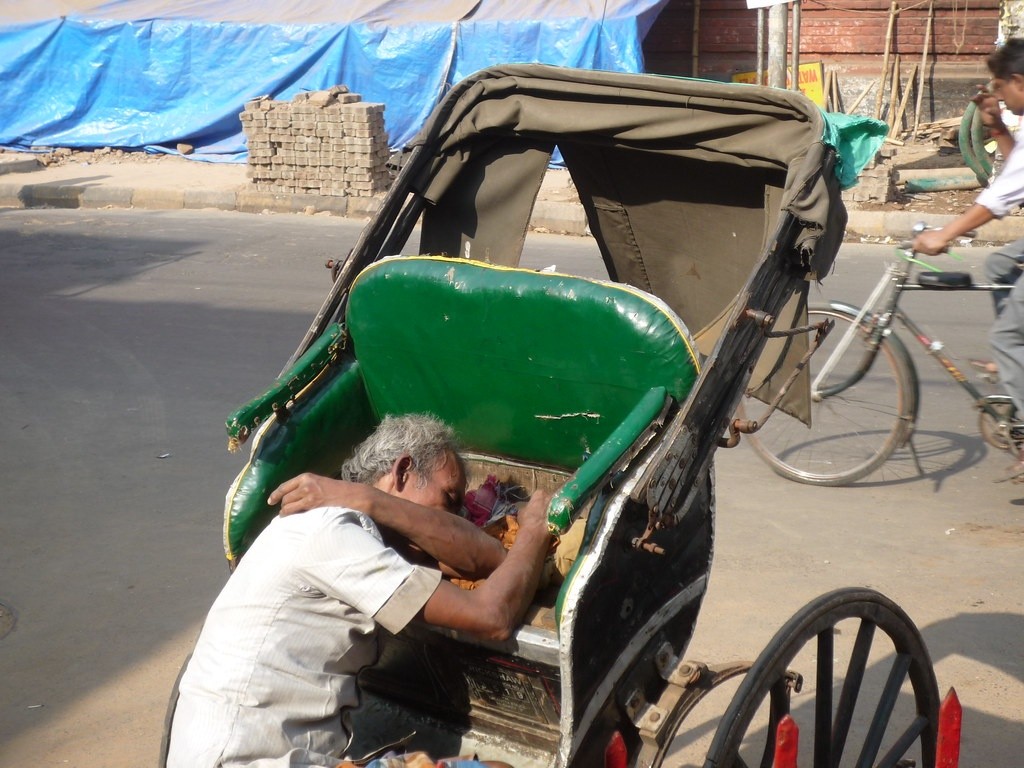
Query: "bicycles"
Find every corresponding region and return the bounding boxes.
[732,221,1024,485]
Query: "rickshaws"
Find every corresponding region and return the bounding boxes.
[158,66,941,768]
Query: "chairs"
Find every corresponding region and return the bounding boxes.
[222,254,703,666]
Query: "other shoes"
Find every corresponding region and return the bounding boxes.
[992,460,1024,483]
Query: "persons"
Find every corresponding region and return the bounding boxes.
[913,38,1024,415]
[164,411,556,768]
